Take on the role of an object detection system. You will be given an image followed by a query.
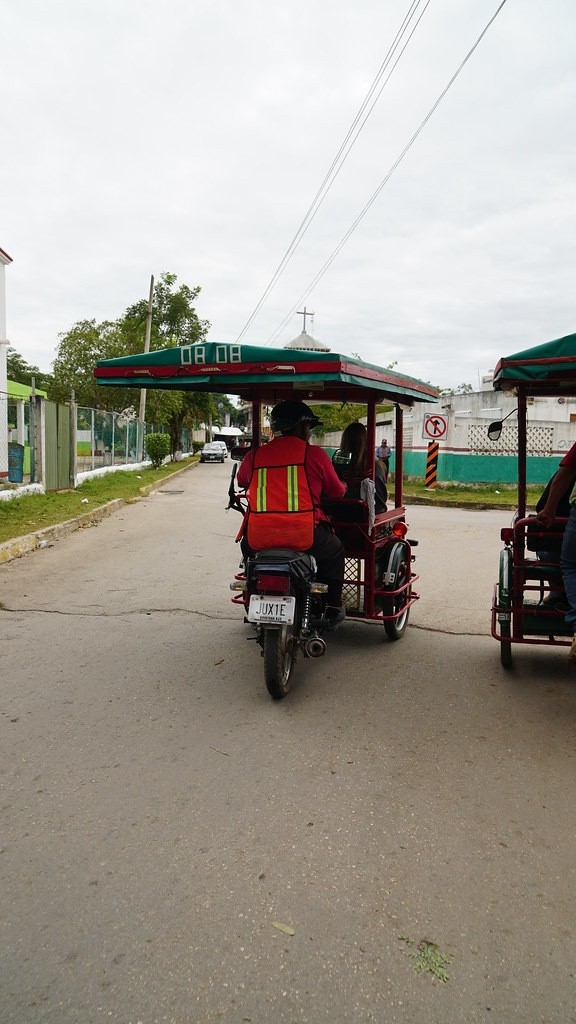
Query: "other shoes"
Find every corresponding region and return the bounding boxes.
[543,593,568,605]
[325,605,345,627]
[568,634,576,665]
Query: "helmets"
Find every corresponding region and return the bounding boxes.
[270,400,320,432]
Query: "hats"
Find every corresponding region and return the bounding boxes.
[382,439,387,444]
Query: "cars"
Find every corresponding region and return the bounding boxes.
[200,443,224,463]
[213,440,227,458]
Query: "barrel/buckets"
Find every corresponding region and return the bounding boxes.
[8,442,25,483]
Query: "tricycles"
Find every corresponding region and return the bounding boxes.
[94,340,439,702]
[488,334,576,671]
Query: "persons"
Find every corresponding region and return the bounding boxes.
[332,423,386,534]
[240,424,244,431]
[237,399,348,633]
[228,437,236,447]
[535,443,576,670]
[377,439,392,480]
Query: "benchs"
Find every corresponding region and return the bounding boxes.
[525,515,569,580]
[323,492,394,548]
